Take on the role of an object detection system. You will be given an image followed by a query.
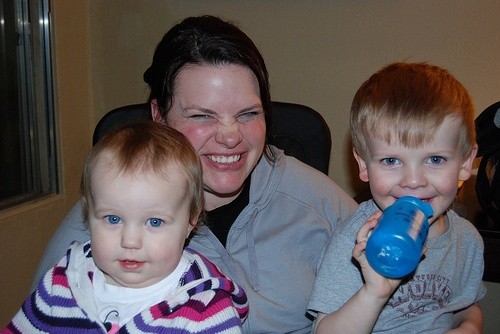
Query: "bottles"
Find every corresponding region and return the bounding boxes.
[365,196,434,279]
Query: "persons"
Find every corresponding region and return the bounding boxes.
[477,101,500,231]
[307,63,487,334]
[32,16,358,334]
[0,121,248,334]
